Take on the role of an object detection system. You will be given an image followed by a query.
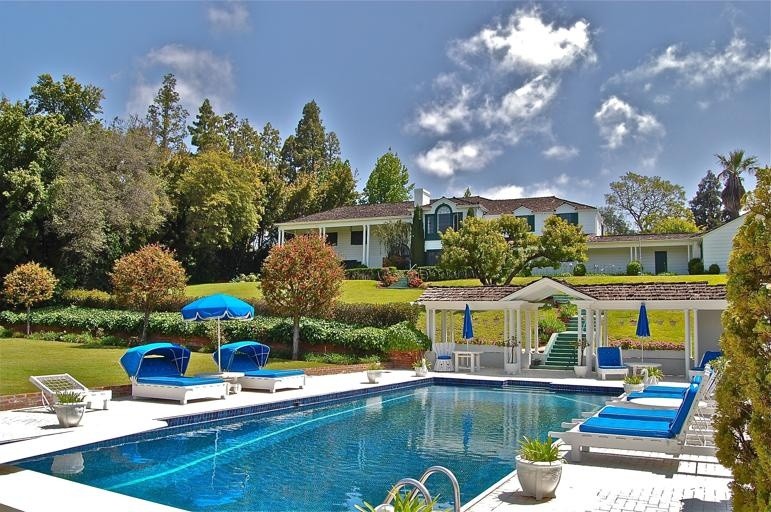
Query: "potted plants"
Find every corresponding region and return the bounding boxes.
[366,354,384,383]
[513,430,570,501]
[51,388,88,429]
[411,358,432,377]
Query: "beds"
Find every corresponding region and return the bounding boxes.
[119,339,308,405]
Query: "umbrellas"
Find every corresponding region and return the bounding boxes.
[179,294,256,370]
[635,300,651,363]
[462,303,474,366]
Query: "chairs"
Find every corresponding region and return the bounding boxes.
[547,347,726,462]
[432,342,456,372]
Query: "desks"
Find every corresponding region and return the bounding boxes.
[452,350,484,373]
